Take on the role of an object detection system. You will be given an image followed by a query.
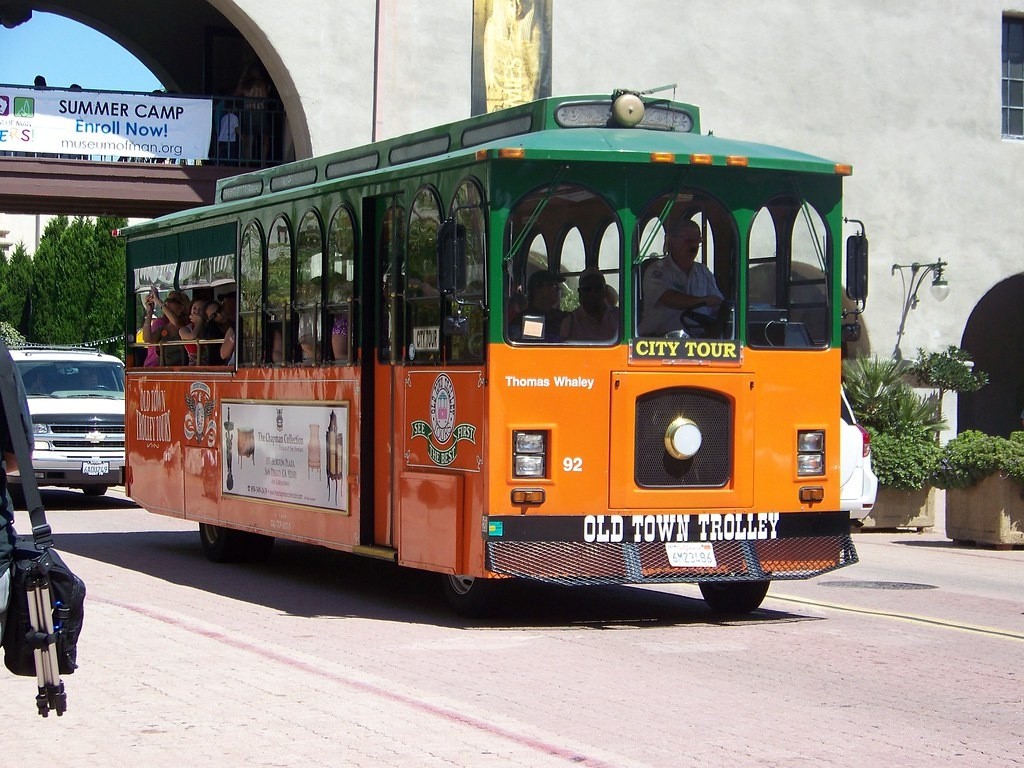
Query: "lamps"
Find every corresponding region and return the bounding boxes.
[892,256,950,304]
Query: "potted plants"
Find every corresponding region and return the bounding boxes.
[841,349,949,533]
[929,429,1024,550]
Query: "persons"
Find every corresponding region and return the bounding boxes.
[219,112,240,166]
[639,218,724,340]
[238,61,276,168]
[142,265,620,363]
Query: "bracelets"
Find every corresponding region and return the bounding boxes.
[144,317,151,320]
[160,304,167,309]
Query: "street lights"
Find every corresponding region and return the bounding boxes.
[888,258,951,372]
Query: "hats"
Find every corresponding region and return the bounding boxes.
[527,270,565,291]
[217,291,236,300]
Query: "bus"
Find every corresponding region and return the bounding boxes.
[120,83,869,616]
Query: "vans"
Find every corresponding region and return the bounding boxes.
[10,347,130,495]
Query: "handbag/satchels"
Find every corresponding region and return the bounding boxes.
[2,537,85,677]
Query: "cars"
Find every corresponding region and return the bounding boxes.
[841,382,880,530]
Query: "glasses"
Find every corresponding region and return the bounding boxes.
[165,297,184,306]
[674,235,704,244]
[581,286,605,292]
[209,306,223,321]
